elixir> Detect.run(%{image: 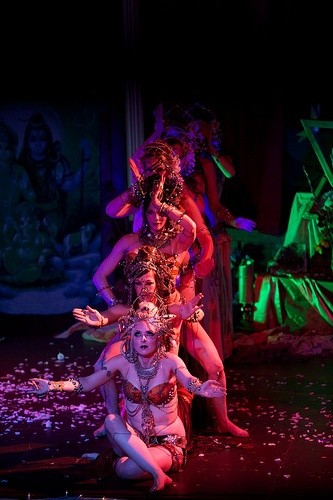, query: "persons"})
[29,305,227,494]
[59,103,248,446]
